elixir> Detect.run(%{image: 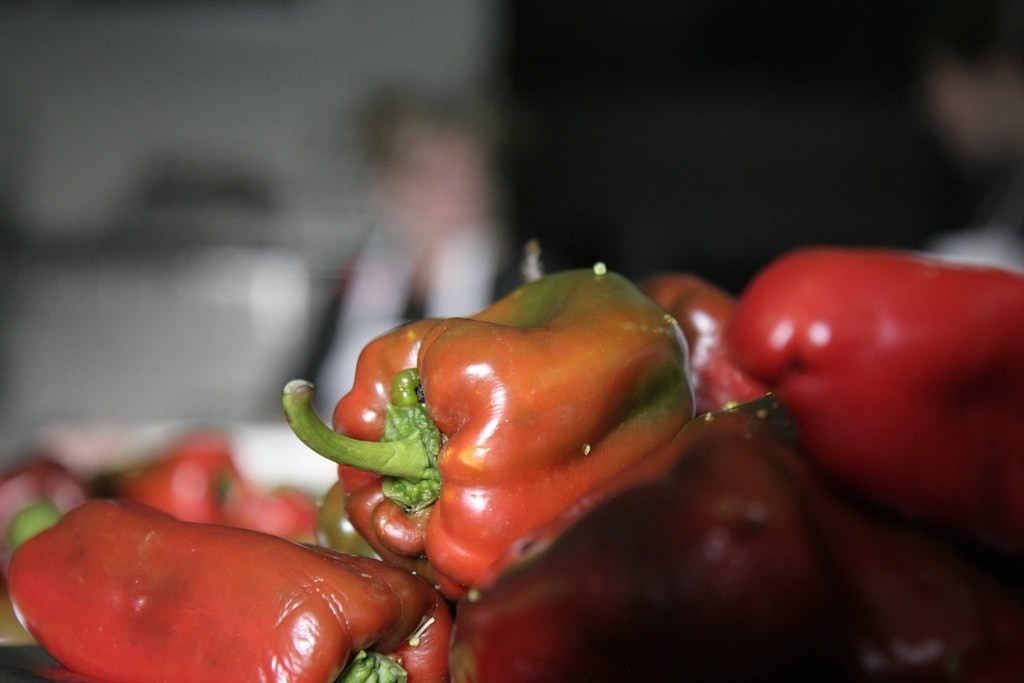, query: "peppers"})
[0,239,1024,683]
